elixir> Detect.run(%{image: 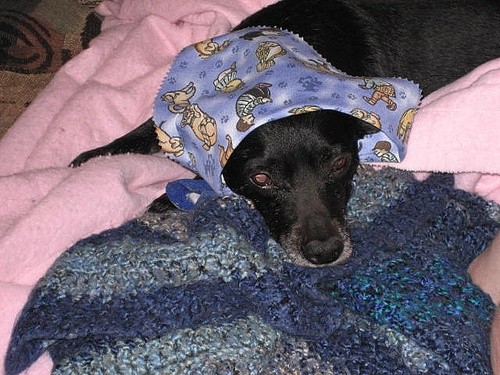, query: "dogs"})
[67,0,500,269]
